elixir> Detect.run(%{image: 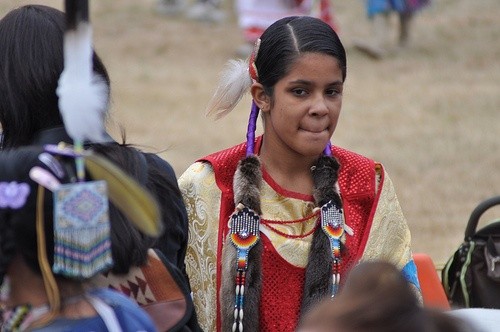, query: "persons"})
[0,3,200,332]
[234,0,430,61]
[177,15,424,332]
